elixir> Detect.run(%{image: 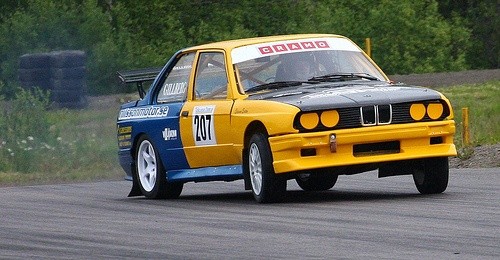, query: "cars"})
[116,34,457,205]
